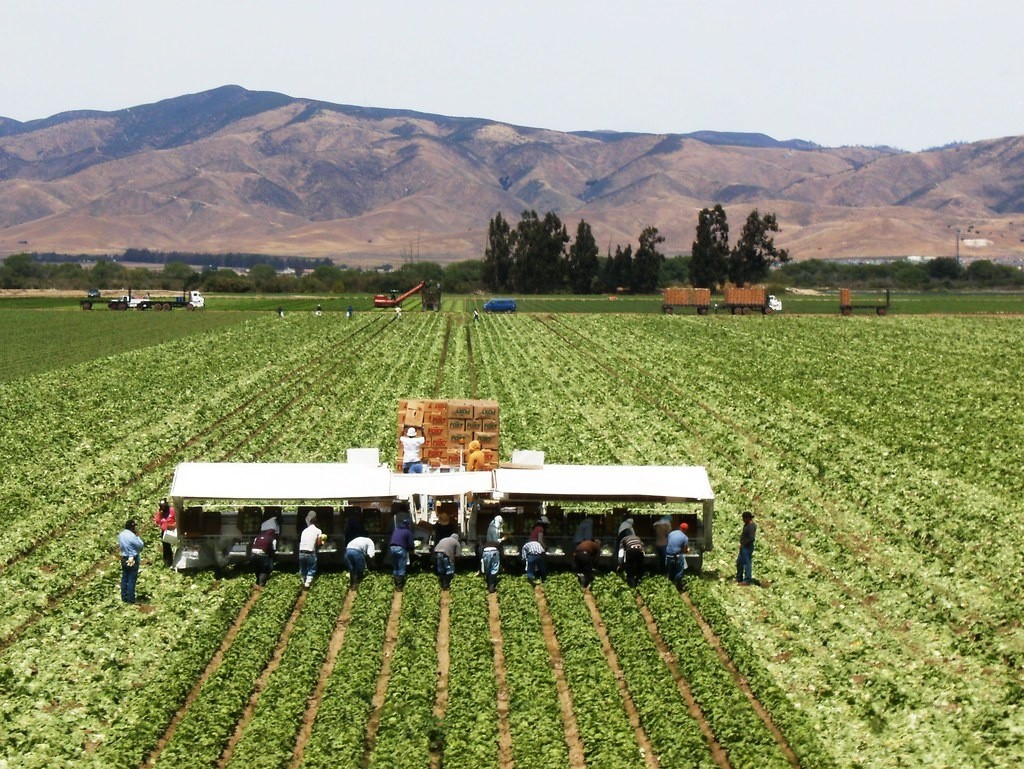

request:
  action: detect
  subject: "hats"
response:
[451,534,459,539]
[158,499,166,505]
[401,519,411,526]
[125,520,138,526]
[266,529,274,535]
[742,512,755,518]
[538,516,550,523]
[309,518,318,524]
[681,523,688,529]
[469,440,480,450]
[406,427,416,436]
[595,540,601,546]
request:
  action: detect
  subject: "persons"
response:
[466,440,486,508]
[394,306,401,317]
[389,520,414,592]
[482,515,508,594]
[472,309,479,323]
[713,304,718,315]
[298,510,322,589]
[734,512,756,586]
[278,304,282,317]
[573,518,601,588]
[616,519,644,589]
[522,516,550,588]
[317,304,322,317]
[652,515,689,591]
[400,427,425,513]
[347,305,352,317]
[155,498,176,569]
[344,537,375,588]
[251,528,277,586]
[118,520,144,604]
[432,513,461,588]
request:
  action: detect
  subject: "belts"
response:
[299,551,313,554]
[578,551,590,556]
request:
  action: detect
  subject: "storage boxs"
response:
[181,399,697,537]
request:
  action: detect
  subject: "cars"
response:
[87,288,101,298]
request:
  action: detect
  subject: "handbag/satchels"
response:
[163,529,177,544]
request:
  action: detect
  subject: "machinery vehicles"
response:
[374,280,425,308]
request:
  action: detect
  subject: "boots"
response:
[252,567,688,594]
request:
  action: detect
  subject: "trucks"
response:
[663,289,783,315]
[422,280,441,311]
[79,286,205,312]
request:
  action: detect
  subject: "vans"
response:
[483,298,516,314]
